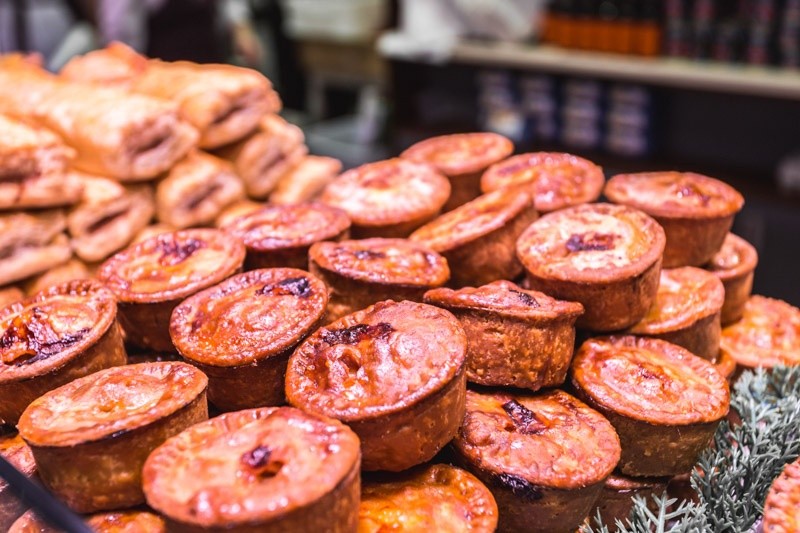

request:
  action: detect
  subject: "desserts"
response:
[0,42,800,533]
